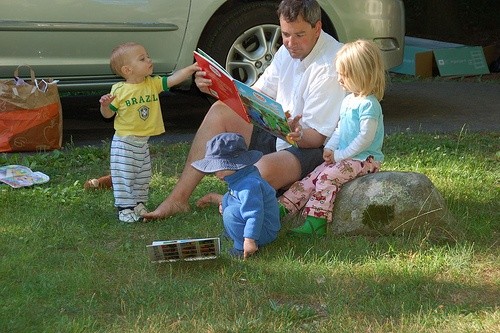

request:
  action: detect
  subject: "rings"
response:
[299,130,304,137]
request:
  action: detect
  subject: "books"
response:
[193,47,300,151]
[0,164,50,188]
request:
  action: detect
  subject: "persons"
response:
[99,40,203,223]
[189,131,281,260]
[275,38,386,237]
[141,0,352,221]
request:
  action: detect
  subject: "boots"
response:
[279,202,286,218]
[286,216,326,238]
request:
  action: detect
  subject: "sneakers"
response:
[118,203,150,222]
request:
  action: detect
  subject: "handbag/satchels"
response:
[0,64,63,153]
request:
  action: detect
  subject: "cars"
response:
[1,1,407,134]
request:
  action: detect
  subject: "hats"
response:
[191,133,263,173]
